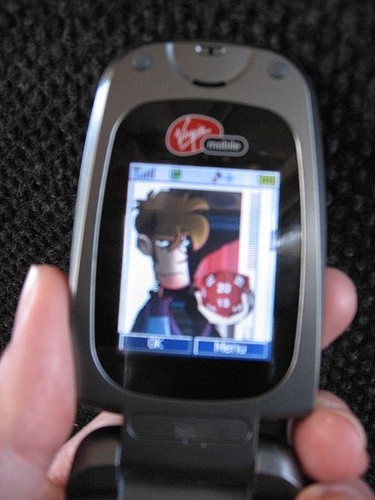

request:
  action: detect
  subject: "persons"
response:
[1,264,375,499]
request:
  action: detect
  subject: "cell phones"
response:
[63,38,328,500]
